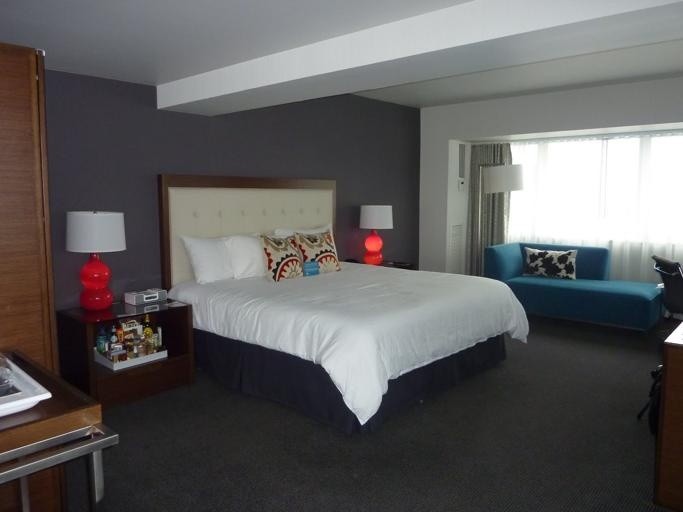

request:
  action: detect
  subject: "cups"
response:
[95,336,107,353]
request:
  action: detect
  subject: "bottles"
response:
[133,314,162,358]
[110,325,118,344]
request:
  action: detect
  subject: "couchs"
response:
[483,242,661,332]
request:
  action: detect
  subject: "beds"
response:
[160,172,509,436]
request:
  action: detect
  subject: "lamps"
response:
[359,205,393,265]
[65,210,127,311]
[477,163,523,272]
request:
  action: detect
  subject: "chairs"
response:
[650,255,683,314]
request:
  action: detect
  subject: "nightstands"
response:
[57,298,194,411]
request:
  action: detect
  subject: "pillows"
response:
[523,247,578,279]
[182,224,341,284]
